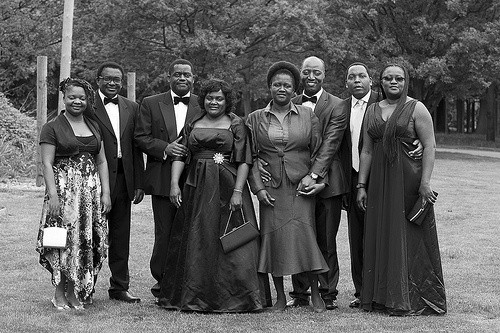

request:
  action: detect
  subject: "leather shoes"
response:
[109,289,140,303]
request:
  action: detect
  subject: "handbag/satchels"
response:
[220,207,259,254]
[43,216,67,248]
[407,191,438,226]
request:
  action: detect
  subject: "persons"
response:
[159,80,263,313]
[35,77,112,310]
[255,56,349,310]
[245,60,329,314]
[86,63,145,304]
[356,64,448,315]
[341,61,383,298]
[134,59,205,302]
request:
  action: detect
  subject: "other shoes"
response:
[321,296,337,310]
[286,298,309,307]
[349,298,361,307]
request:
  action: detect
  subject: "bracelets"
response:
[234,189,242,193]
[356,183,366,188]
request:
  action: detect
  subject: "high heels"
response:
[51,298,70,311]
[272,301,286,313]
[66,295,84,311]
[312,294,325,313]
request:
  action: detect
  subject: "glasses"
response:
[99,77,123,84]
[381,76,405,82]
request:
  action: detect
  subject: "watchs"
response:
[308,172,319,179]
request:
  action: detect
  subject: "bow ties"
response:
[104,97,118,106]
[173,97,190,105]
[302,95,318,104]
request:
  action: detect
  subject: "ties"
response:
[352,100,364,173]
